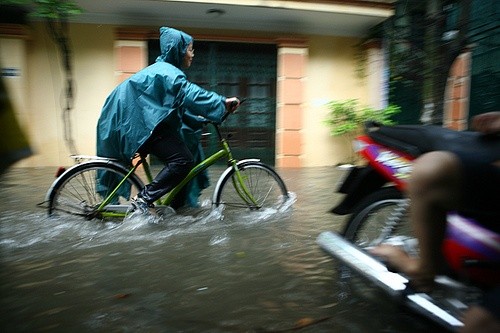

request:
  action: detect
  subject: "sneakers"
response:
[131,194,163,218]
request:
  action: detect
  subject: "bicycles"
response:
[34,96,297,231]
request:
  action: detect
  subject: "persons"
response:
[456,267,500,332]
[94,26,241,233]
[374,112,500,287]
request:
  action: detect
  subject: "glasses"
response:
[190,49,194,54]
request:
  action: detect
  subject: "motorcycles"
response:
[321,120,500,333]
